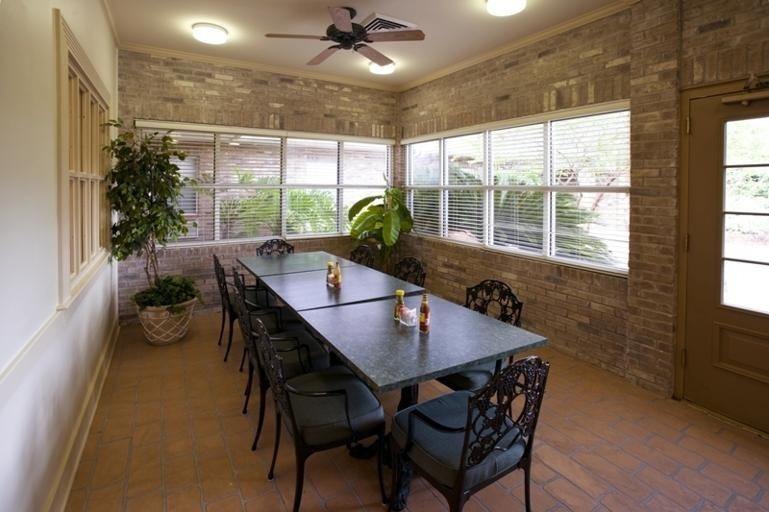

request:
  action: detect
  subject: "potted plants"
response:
[99,117,205,346]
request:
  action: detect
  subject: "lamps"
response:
[192,22,228,44]
[486,0,526,17]
[369,61,396,75]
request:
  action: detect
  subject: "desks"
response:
[235,250,550,414]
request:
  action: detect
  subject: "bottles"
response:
[334,262,342,288]
[393,289,405,320]
[418,294,430,334]
[326,262,335,287]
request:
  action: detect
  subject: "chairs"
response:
[432,278,524,420]
[349,244,375,270]
[389,355,550,510]
[254,316,390,511]
[255,239,295,256]
[390,255,427,288]
[214,254,332,452]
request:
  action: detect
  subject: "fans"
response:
[265,6,425,67]
